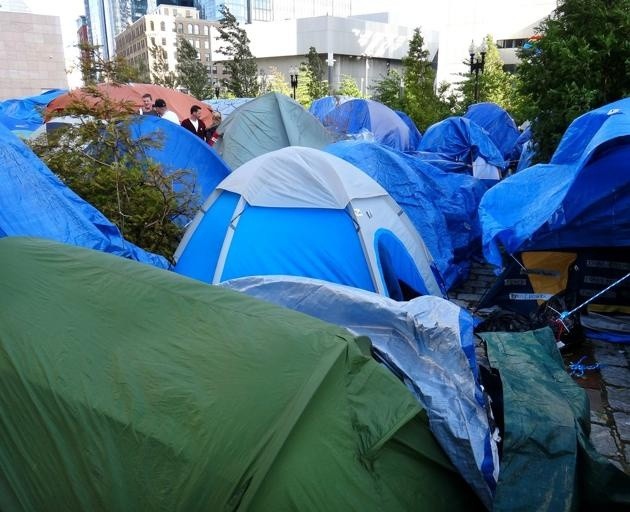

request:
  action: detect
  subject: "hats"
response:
[152,99,167,108]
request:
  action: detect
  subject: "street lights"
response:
[214,80,221,100]
[290,63,298,100]
[470,37,488,103]
